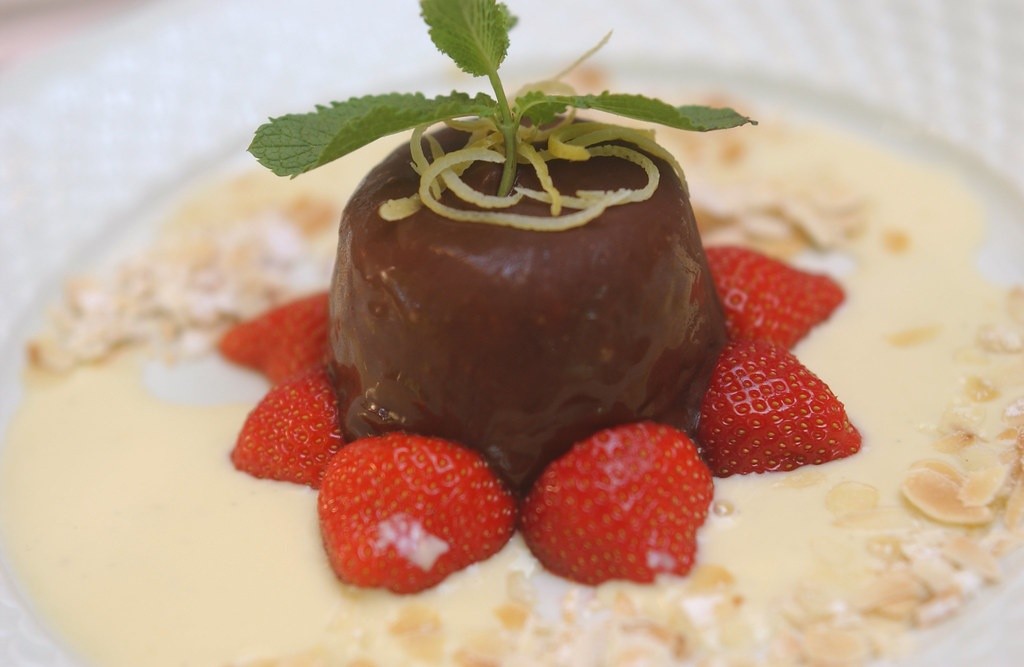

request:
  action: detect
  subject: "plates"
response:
[0,0,1024,667]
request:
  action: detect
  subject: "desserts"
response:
[11,69,1024,667]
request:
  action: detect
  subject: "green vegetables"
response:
[249,0,758,200]
